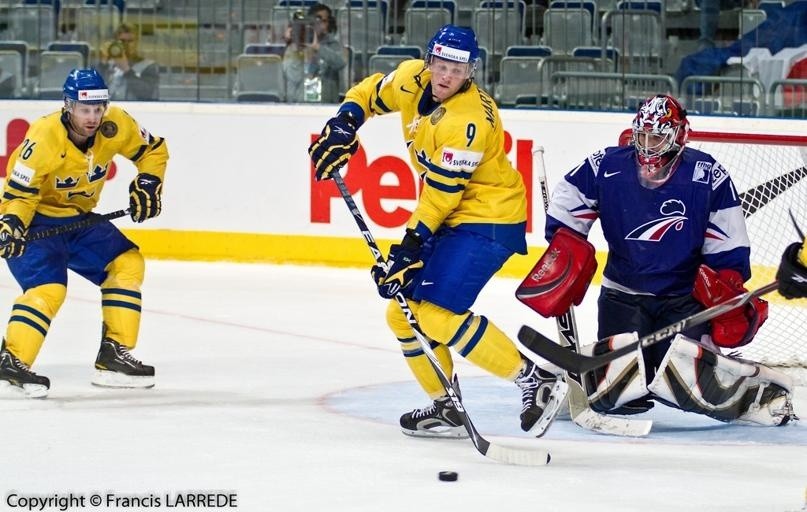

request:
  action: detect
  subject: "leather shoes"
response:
[368,244,427,298]
[128,172,164,223]
[0,214,30,260]
[307,110,362,185]
[776,239,807,302]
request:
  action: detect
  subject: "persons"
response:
[282,3,348,104]
[0,66,169,390]
[515,95,794,428]
[97,25,160,102]
[309,23,556,432]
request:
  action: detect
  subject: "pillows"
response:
[421,21,481,82]
[627,93,692,192]
[62,67,111,110]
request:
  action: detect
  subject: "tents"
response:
[325,5,337,33]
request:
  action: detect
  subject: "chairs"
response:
[510,349,559,433]
[94,320,155,377]
[396,372,467,431]
[0,0,807,119]
[0,333,51,391]
